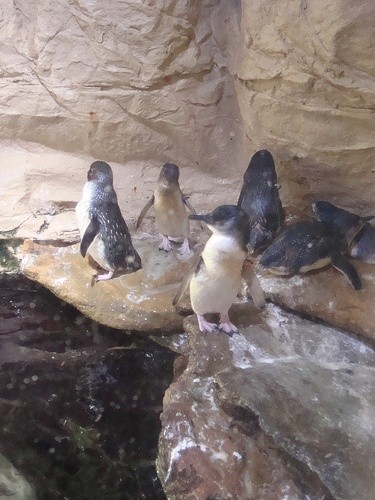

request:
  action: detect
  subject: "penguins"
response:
[173,204,267,335]
[235,148,287,257]
[74,160,144,288]
[309,200,375,264]
[134,162,204,257]
[253,219,362,292]
